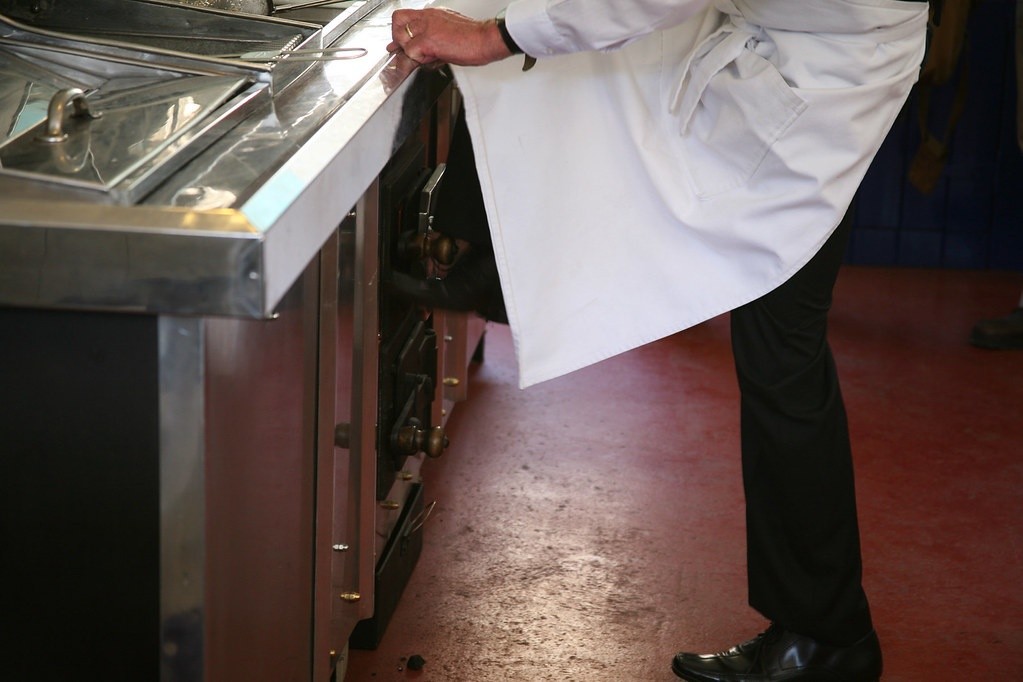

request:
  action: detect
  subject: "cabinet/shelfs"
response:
[208,78,489,682]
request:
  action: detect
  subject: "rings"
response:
[404,22,414,39]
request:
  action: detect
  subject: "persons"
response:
[969,296,1023,349]
[385,1,930,682]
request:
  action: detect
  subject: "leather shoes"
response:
[671,622,882,682]
[383,244,508,324]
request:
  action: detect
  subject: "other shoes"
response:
[969,307,1023,352]
